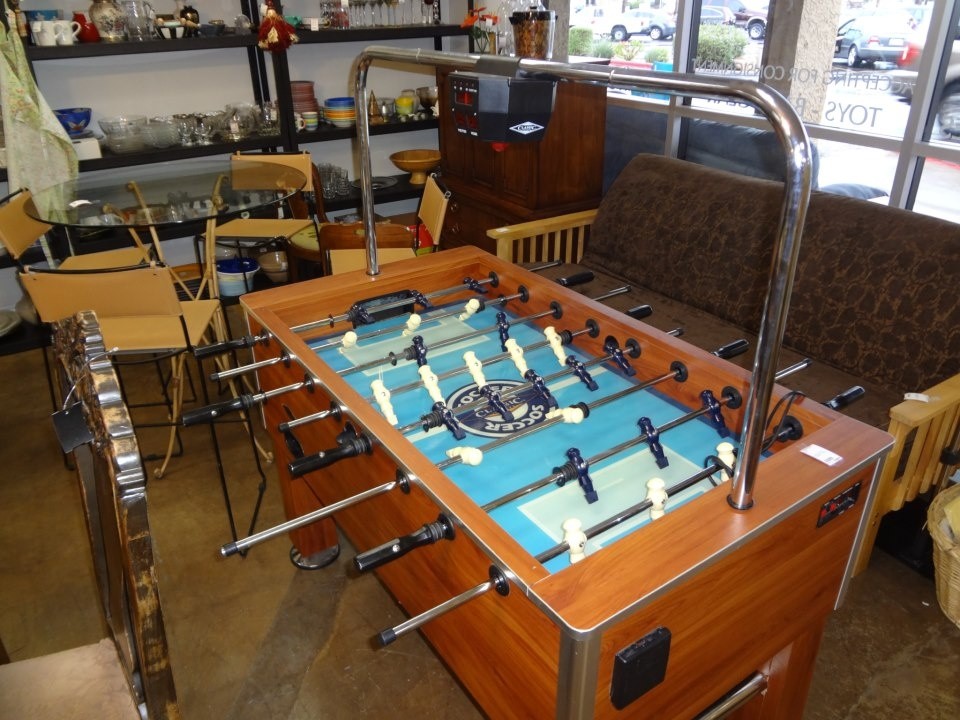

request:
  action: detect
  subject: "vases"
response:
[217,258,261,297]
[259,252,288,283]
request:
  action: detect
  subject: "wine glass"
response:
[348,0,434,28]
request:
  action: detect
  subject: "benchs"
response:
[487,152,960,577]
[602,106,888,200]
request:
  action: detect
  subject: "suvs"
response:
[701,0,768,41]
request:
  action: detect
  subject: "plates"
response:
[351,176,398,189]
[290,80,320,122]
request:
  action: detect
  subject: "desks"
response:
[24,160,307,479]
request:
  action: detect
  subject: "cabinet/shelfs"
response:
[434,66,607,264]
[22,0,474,308]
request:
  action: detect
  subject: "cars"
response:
[570,5,676,42]
[832,15,914,69]
[882,25,960,139]
[698,6,737,26]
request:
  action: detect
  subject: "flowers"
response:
[460,3,500,52]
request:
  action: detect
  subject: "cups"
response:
[318,162,350,199]
[79,214,124,230]
[293,112,305,133]
[302,112,317,130]
[121,0,150,38]
[320,0,350,30]
[54,20,80,45]
[29,21,63,46]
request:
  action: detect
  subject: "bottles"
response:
[89,0,125,39]
[496,0,546,57]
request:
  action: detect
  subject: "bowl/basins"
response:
[153,26,189,39]
[52,107,92,135]
[323,97,356,128]
[213,243,288,296]
[196,24,225,36]
[97,115,147,137]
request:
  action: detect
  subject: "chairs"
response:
[0,150,451,720]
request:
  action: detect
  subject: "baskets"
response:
[927,483,960,626]
